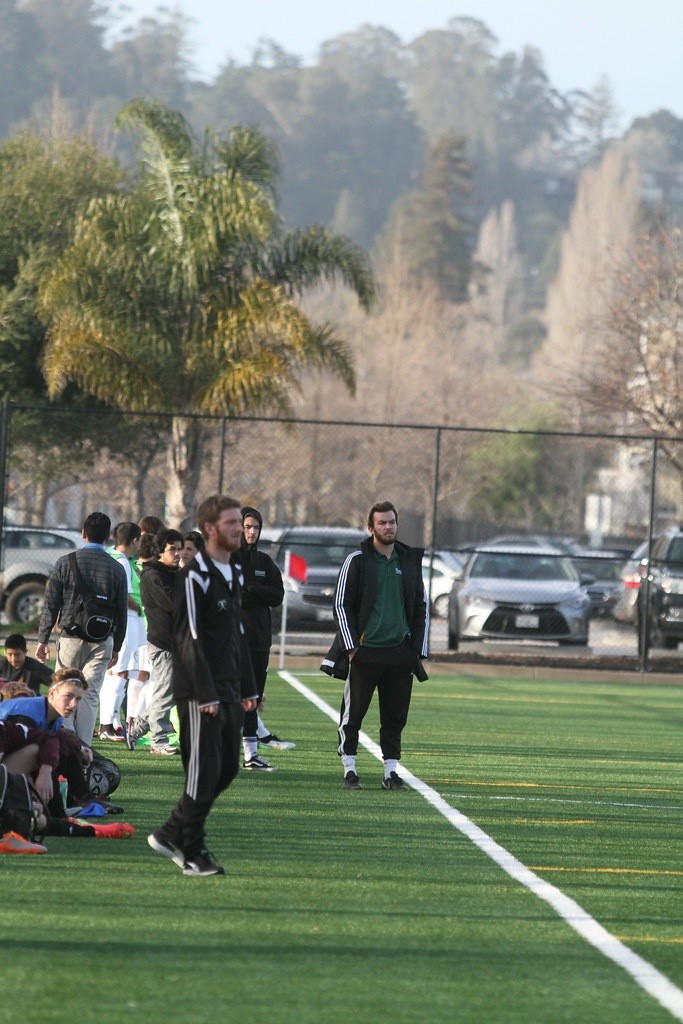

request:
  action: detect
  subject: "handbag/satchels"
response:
[70,587,117,643]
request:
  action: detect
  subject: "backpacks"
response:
[0,764,50,845]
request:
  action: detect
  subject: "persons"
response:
[256,716,297,750]
[93,521,146,742]
[36,511,127,748]
[228,506,286,771]
[146,496,259,875]
[0,634,135,854]
[320,502,429,792]
[129,516,204,755]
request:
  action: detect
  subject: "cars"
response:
[258,525,375,637]
[0,525,99,635]
[447,545,594,647]
[418,531,661,621]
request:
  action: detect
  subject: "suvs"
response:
[636,526,683,657]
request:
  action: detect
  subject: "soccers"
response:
[84,767,109,798]
[91,757,121,796]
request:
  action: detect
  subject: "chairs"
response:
[480,560,500,576]
[532,565,560,578]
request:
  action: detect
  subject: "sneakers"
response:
[242,755,277,771]
[99,729,125,742]
[262,735,296,750]
[344,770,363,789]
[149,826,184,869]
[182,848,225,875]
[85,823,135,839]
[382,771,409,790]
[0,831,49,854]
[126,716,137,750]
[105,806,124,814]
[149,745,180,755]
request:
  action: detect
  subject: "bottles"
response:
[58,775,68,809]
[30,809,38,842]
